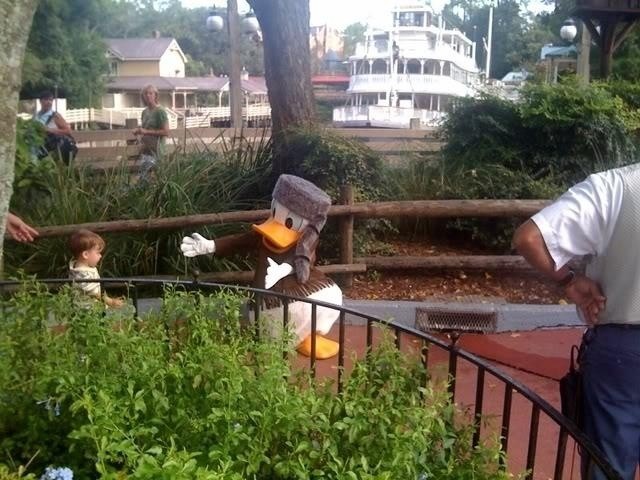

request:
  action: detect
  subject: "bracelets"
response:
[555,267,576,288]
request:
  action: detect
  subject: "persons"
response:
[6,211,39,243]
[512,160,640,479]
[30,89,71,158]
[132,83,170,189]
[65,229,125,318]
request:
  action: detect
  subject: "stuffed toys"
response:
[179,173,344,359]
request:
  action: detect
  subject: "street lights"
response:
[207,1,261,138]
[561,17,602,87]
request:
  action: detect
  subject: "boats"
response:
[332,4,523,130]
